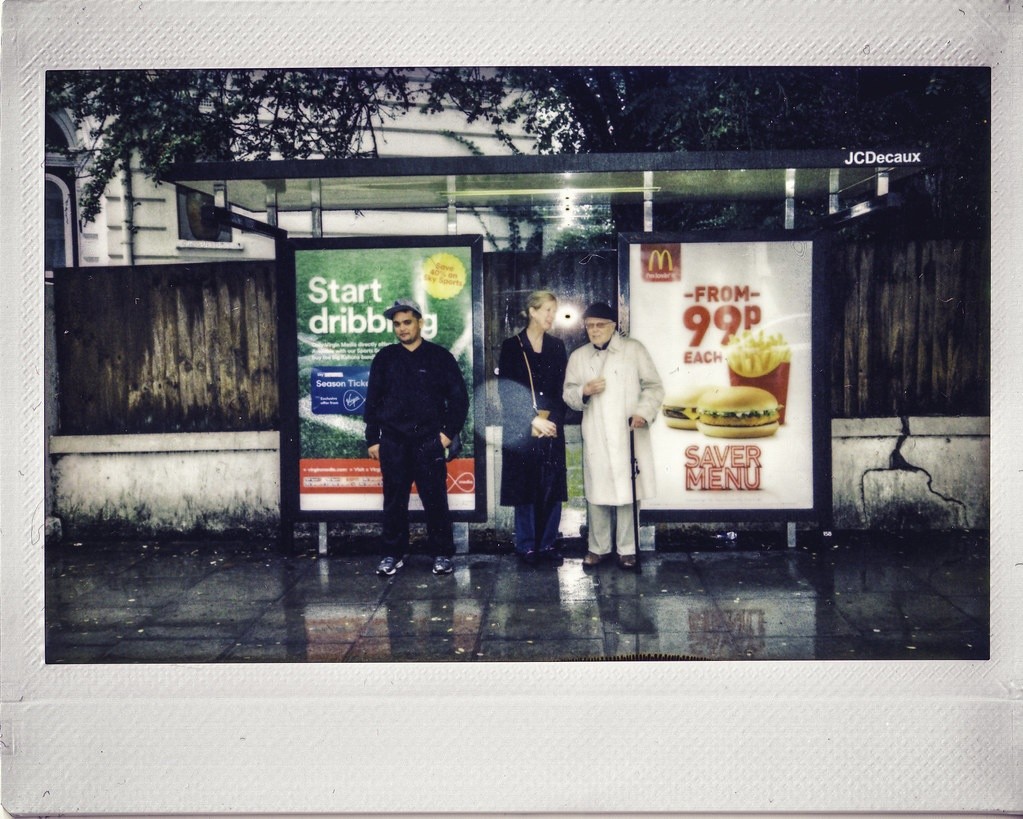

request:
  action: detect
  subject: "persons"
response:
[498,291,583,564]
[563,302,665,568]
[363,297,470,576]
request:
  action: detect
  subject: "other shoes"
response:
[523,547,563,566]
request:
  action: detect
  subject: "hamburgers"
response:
[695,385,783,438]
[661,385,716,430]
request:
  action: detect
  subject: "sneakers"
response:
[376,555,404,577]
[432,556,455,574]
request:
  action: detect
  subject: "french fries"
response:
[726,328,790,378]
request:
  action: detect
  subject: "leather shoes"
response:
[582,552,607,567]
[620,555,636,568]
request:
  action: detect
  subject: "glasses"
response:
[394,318,416,326]
[584,322,615,329]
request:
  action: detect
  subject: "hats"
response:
[383,297,423,320]
[584,302,617,321]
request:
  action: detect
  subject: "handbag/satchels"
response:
[529,408,550,437]
